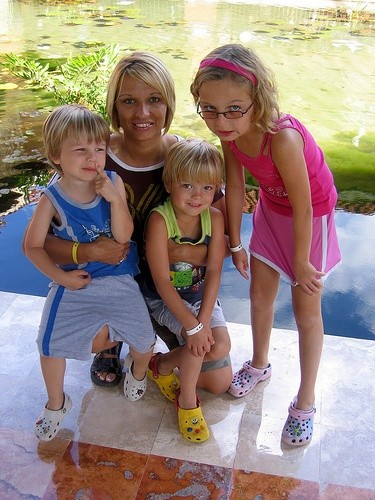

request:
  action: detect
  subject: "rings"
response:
[120,257,125,263]
[126,250,128,256]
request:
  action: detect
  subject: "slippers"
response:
[174,387,210,444]
[281,394,317,447]
[123,352,148,403]
[89,341,123,387]
[144,351,183,403]
[33,392,73,442]
[226,359,273,398]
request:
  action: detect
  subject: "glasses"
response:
[195,94,258,120]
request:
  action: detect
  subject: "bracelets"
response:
[227,241,243,253]
[185,322,204,336]
[72,242,84,265]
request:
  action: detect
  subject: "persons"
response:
[24,105,158,442]
[145,138,231,443]
[23,52,231,394]
[189,43,342,447]
